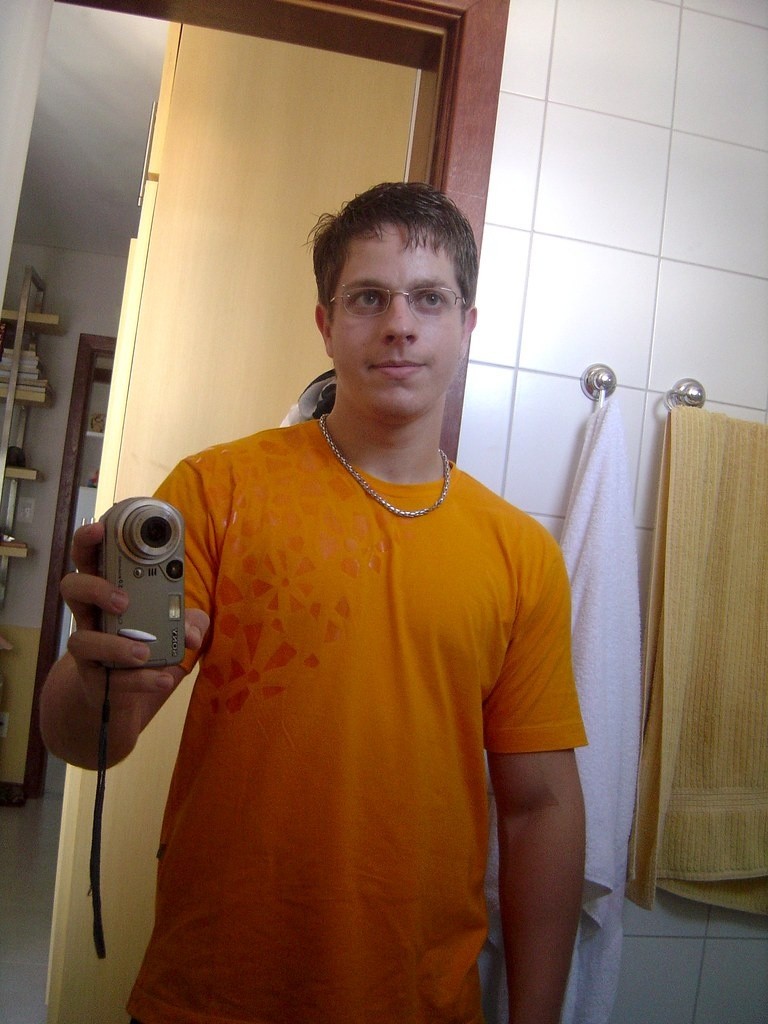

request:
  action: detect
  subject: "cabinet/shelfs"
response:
[0,263,63,609]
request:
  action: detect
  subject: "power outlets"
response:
[0,711,9,738]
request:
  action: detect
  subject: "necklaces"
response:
[320,412,451,518]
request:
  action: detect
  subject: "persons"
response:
[40,184,589,1024]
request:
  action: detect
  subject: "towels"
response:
[473,385,641,1024]
[624,399,768,928]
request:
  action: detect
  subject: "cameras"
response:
[97,495,188,668]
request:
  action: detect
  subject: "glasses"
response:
[329,286,466,319]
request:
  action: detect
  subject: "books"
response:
[0,348,54,393]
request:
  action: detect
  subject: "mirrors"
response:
[0,0,768,1024]
